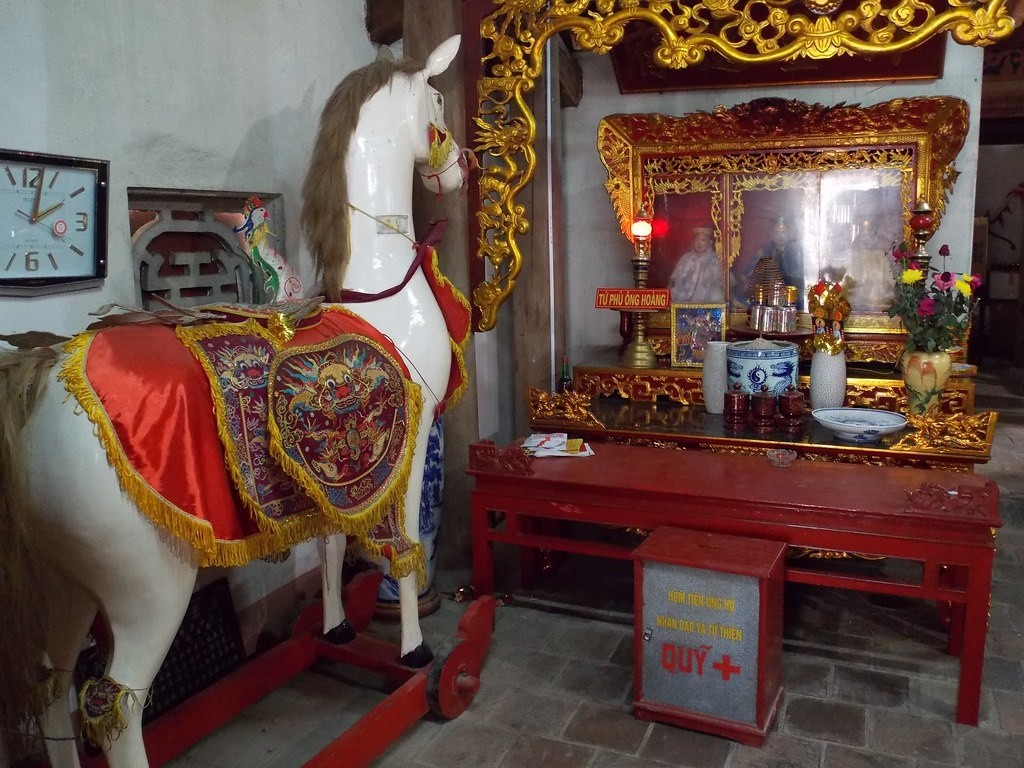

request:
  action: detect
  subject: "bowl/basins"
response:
[812,407,908,443]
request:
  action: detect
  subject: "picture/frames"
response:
[671,304,726,367]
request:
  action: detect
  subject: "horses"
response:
[0,33,469,767]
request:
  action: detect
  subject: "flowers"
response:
[884,239,981,353]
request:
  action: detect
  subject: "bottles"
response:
[558,356,573,394]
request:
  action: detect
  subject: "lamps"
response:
[621,204,657,368]
[908,194,934,293]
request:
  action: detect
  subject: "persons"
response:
[667,215,898,311]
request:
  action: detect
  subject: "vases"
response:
[810,349,845,410]
[900,349,951,415]
[701,341,730,414]
[374,416,444,621]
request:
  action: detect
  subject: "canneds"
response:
[750,282,797,333]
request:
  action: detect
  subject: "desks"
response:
[465,348,1003,726]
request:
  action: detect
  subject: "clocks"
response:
[0,149,110,297]
